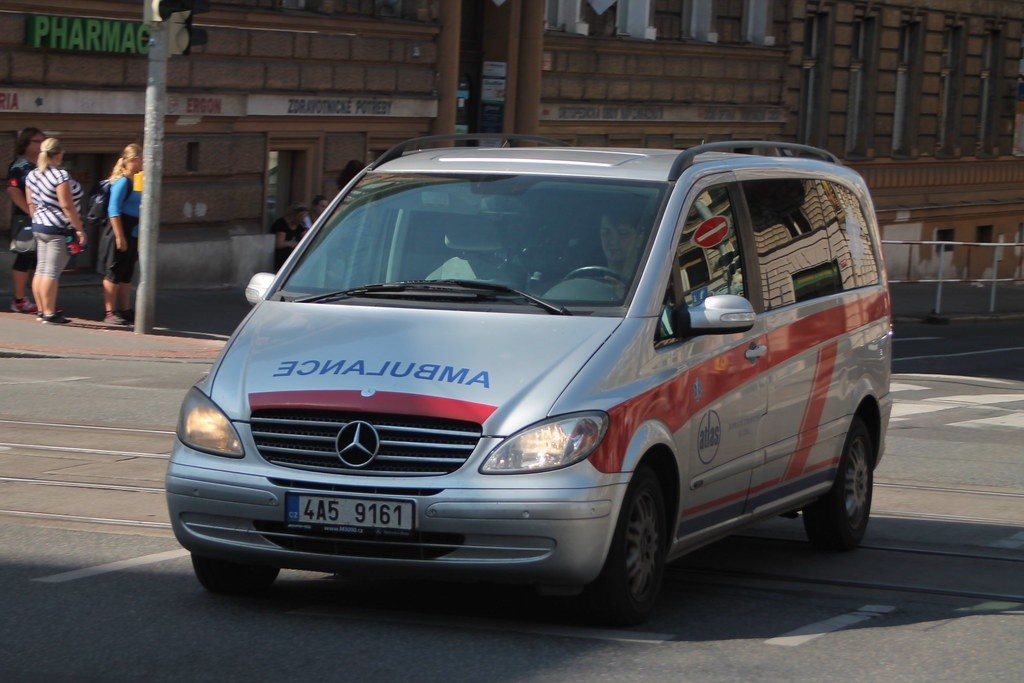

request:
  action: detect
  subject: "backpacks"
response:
[86,176,133,225]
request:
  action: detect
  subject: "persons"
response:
[6,127,143,325]
[599,199,646,298]
[269,195,329,272]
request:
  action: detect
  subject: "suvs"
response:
[163,132,893,626]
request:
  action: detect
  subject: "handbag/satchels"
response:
[9,215,36,252]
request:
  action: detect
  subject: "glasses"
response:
[30,139,43,143]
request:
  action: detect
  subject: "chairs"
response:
[423,220,526,291]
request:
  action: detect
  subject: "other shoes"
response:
[104,311,128,325]
[119,309,134,321]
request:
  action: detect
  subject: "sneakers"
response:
[11,296,37,313]
[56,306,64,312]
[43,310,71,325]
[36,313,44,321]
